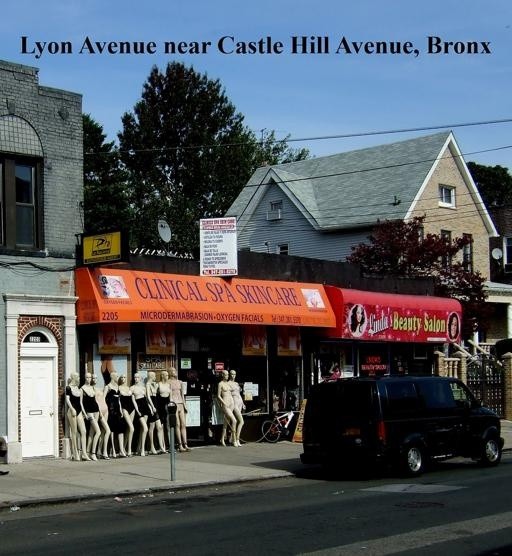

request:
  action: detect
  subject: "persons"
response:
[447,312,460,339]
[229,370,246,447]
[101,276,107,283]
[350,304,366,333]
[216,370,239,448]
[64,367,191,462]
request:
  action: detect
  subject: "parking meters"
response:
[168,401,180,481]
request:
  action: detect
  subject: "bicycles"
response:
[257,394,298,441]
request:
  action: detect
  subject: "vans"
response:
[300,371,507,478]
